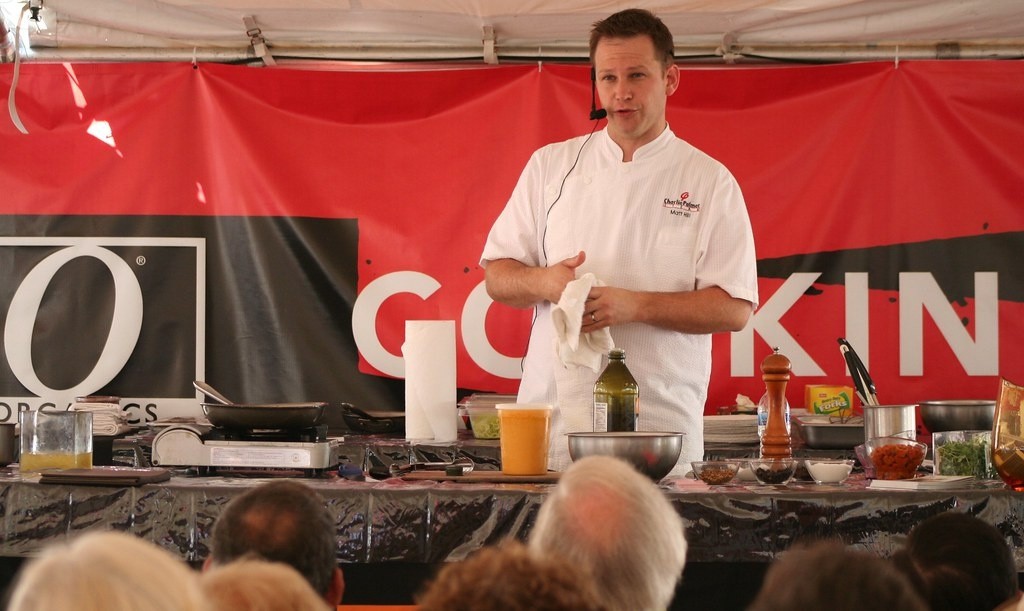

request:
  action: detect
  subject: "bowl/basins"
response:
[564,430,684,487]
[854,436,927,481]
[461,392,518,439]
[748,460,798,485]
[803,460,856,485]
[690,461,742,485]
[726,458,759,481]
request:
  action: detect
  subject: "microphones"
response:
[590,67,607,121]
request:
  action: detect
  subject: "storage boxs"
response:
[933,431,996,479]
[459,408,501,440]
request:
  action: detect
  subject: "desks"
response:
[0,467,1024,610]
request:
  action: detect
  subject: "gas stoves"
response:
[150,420,340,477]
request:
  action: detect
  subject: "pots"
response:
[918,400,996,434]
[338,401,404,435]
[199,401,330,432]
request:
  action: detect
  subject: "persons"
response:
[480,10,761,483]
[895,510,1024,611]
[424,535,614,611]
[4,525,213,611]
[200,478,345,611]
[746,537,929,611]
[527,449,688,611]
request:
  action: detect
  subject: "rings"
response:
[591,313,596,322]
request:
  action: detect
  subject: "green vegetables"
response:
[936,437,998,480]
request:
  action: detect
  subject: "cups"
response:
[19,409,93,472]
[931,430,991,476]
[991,377,1024,491]
[0,422,16,464]
[859,404,919,443]
[496,403,555,477]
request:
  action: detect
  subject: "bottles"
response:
[757,383,792,463]
[593,348,641,434]
